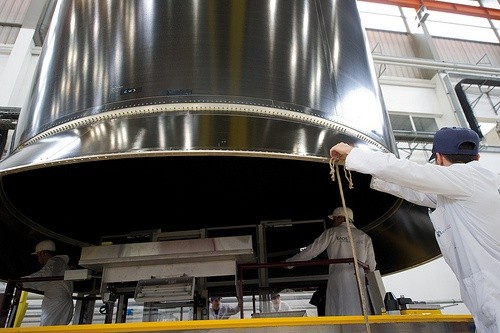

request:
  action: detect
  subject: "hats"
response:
[30,239,56,255]
[429,126,480,162]
[210,292,222,301]
[328,207,355,222]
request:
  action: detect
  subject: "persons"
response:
[282,207,376,316]
[264,293,291,313]
[21,240,73,326]
[329,127,500,333]
[209,296,240,320]
